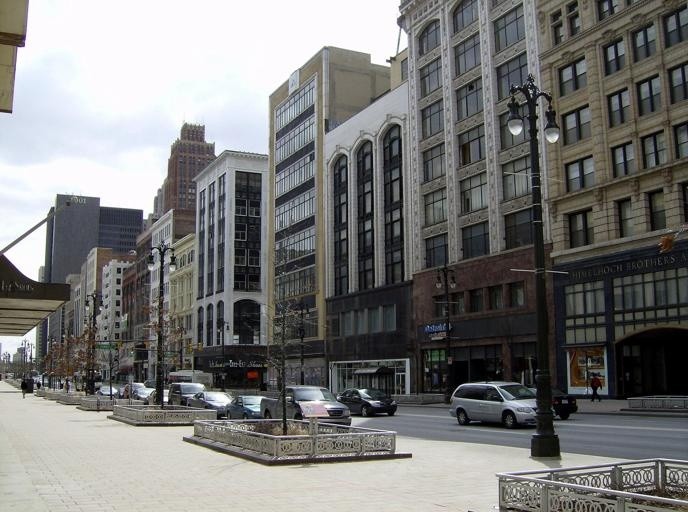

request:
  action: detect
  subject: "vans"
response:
[167,383,207,406]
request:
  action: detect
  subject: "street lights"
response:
[217,320,232,393]
[62,327,75,393]
[505,73,561,458]
[436,265,457,406]
[47,336,56,389]
[147,240,177,405]
[294,301,311,386]
[21,338,35,379]
[84,290,104,396]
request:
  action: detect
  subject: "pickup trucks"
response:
[260,383,351,434]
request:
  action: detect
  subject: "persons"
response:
[122,385,129,399]
[589,374,602,402]
[21,378,28,398]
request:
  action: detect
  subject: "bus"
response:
[75,374,102,391]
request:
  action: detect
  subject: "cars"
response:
[225,395,266,419]
[95,385,118,396]
[336,386,398,417]
[449,382,578,429]
[117,379,169,405]
[186,391,233,420]
